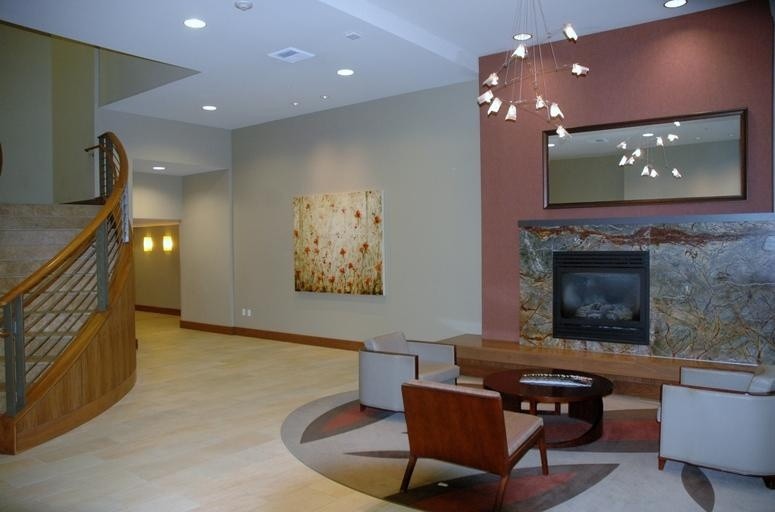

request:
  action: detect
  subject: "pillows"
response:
[747,365,775,392]
[363,331,410,354]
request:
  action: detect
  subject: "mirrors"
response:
[542,108,747,208]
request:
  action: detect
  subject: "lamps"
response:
[615,121,683,179]
[478,0,589,137]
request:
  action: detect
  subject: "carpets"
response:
[281,382,775,512]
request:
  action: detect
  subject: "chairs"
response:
[359,331,460,412]
[400,380,549,512]
[656,365,775,489]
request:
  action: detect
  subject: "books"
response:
[519,373,594,388]
